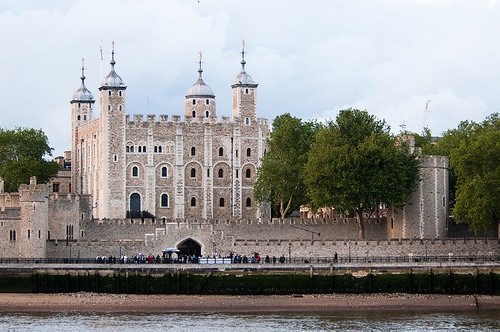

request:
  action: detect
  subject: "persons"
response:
[94,250,339,264]
